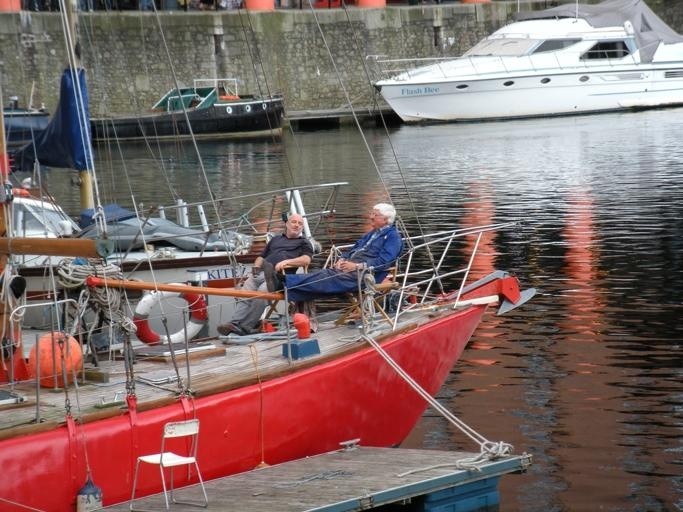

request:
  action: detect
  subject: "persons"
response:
[262,203,403,302]
[216,213,313,336]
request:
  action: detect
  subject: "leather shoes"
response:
[263,259,279,292]
[217,323,246,336]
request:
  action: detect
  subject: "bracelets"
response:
[355,263,359,270]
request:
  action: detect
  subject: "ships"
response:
[1,181,348,331]
[1,264,534,511]
[372,0,682,122]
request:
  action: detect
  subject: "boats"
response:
[77,76,283,143]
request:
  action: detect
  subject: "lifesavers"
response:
[13,188,30,197]
[134,283,207,344]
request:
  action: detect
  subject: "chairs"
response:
[251,259,308,320]
[332,258,400,327]
[128,420,210,512]
